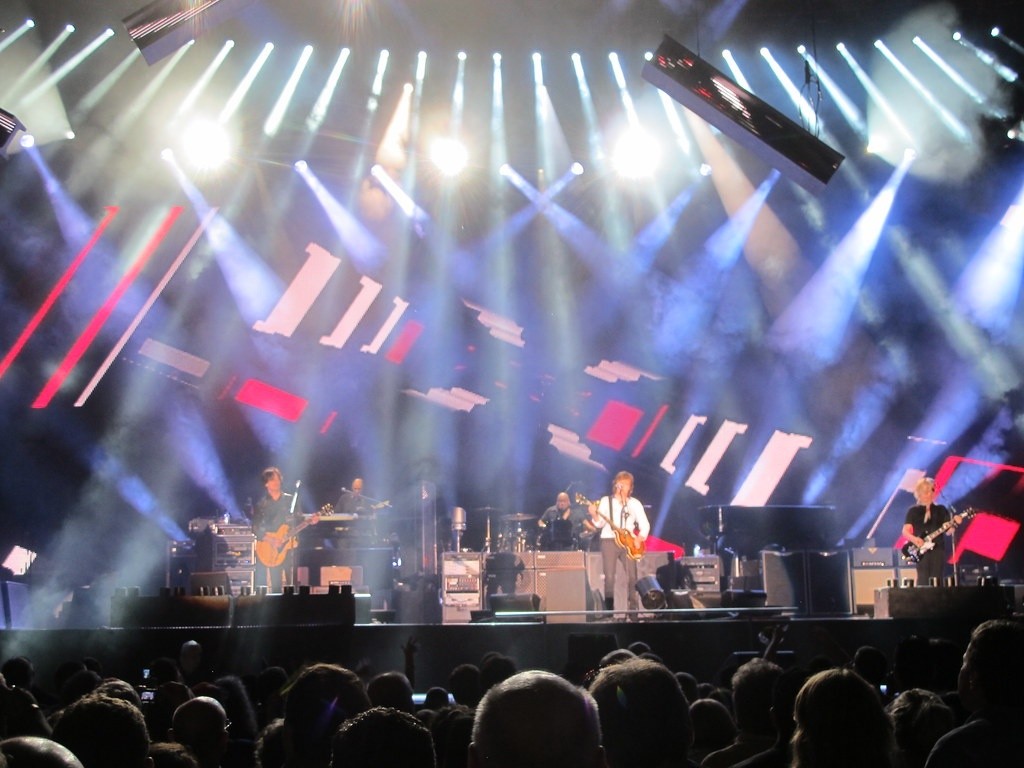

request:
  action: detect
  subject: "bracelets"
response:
[543,525,546,527]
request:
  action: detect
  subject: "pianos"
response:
[698,503,840,617]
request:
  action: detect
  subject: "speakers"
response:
[190,572,232,595]
[481,551,675,624]
[758,549,1015,623]
[319,566,364,588]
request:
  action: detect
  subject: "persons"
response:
[539,493,596,551]
[902,478,963,585]
[0,615,1024,768]
[336,478,368,513]
[589,471,651,621]
[253,466,317,594]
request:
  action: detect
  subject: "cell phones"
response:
[144,668,150,679]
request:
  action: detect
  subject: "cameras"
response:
[136,689,158,705]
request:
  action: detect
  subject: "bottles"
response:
[224,510,230,524]
[694,545,700,557]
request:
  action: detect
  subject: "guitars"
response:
[254,501,335,568]
[901,505,977,567]
[572,489,646,560]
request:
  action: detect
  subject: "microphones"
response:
[616,483,623,490]
[341,487,345,491]
[296,480,302,489]
[932,484,936,491]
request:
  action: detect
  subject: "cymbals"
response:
[474,507,502,512]
[505,514,537,522]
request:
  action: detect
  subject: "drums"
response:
[548,519,573,543]
